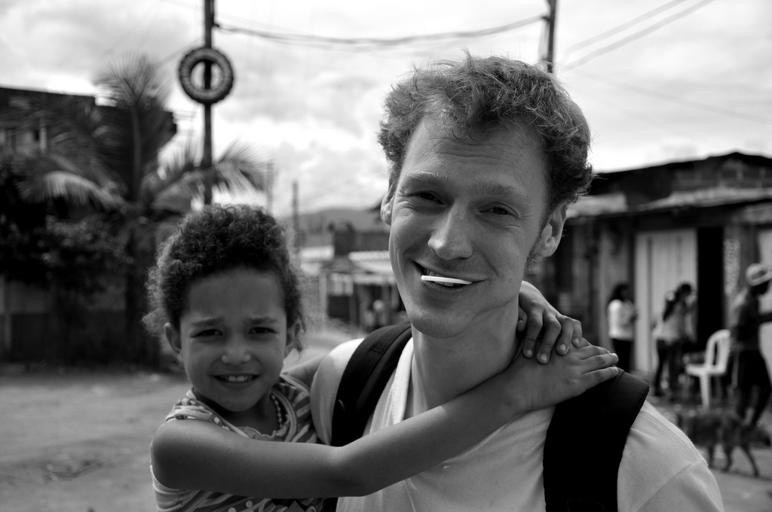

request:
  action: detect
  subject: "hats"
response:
[747,263,771,287]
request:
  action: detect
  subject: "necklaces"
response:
[271,394,283,426]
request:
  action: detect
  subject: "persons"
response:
[309,55,722,510]
[727,262,772,428]
[141,202,624,512]
[606,283,636,374]
[653,283,698,397]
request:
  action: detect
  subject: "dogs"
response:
[672,406,761,477]
